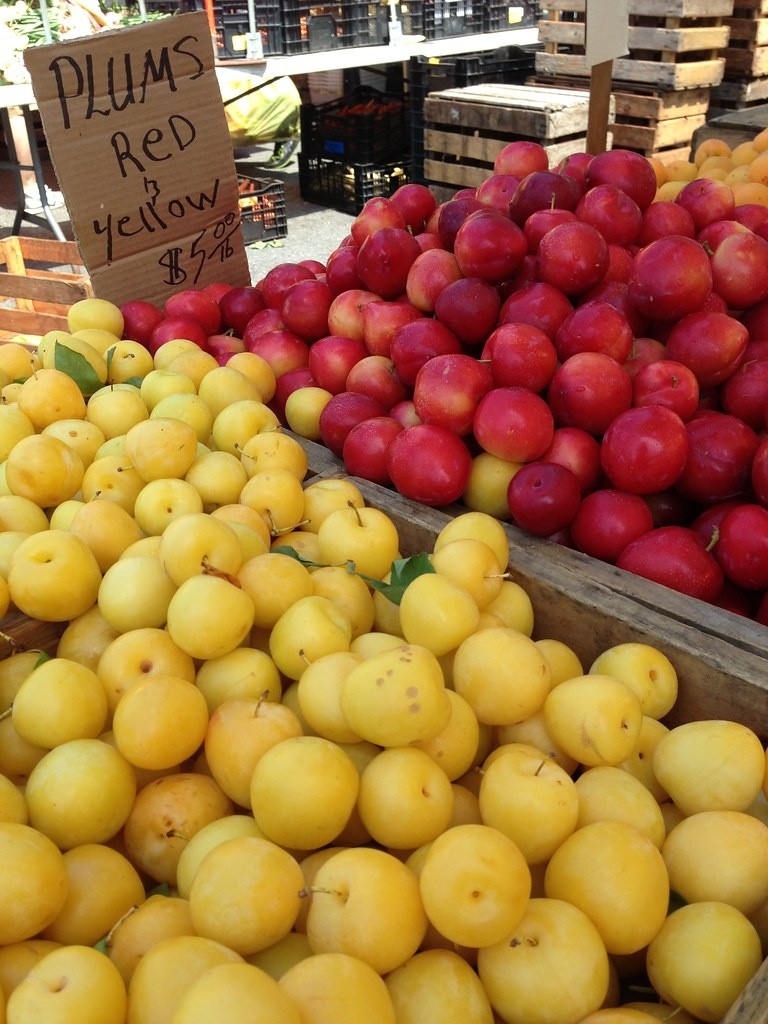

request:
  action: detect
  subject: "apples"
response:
[0,296,768,1024]
[112,126,768,621]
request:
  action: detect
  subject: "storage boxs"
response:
[423,81,615,207]
[533,0,734,93]
[236,173,288,246]
[0,237,94,353]
[395,0,485,43]
[405,45,537,131]
[409,127,504,189]
[524,73,710,166]
[484,0,540,34]
[297,152,416,217]
[280,0,391,56]
[681,0,768,109]
[299,85,410,164]
[124,0,284,60]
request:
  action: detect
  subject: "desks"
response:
[0,84,81,274]
